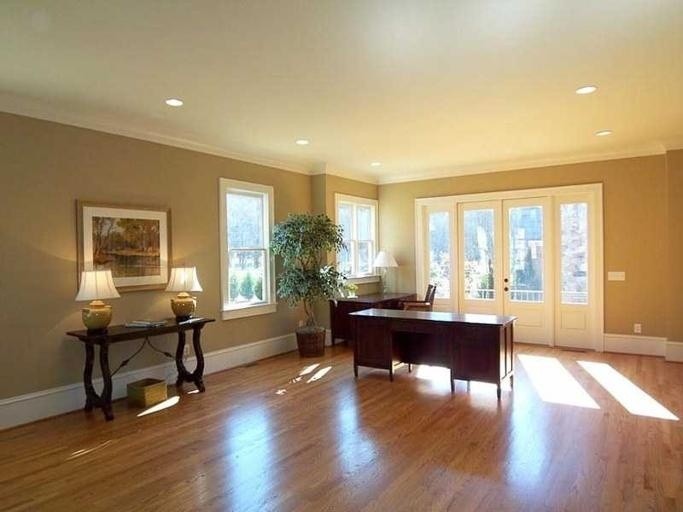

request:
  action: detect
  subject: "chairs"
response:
[398,284,436,311]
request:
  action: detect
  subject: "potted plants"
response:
[268,212,349,357]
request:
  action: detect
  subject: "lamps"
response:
[165,265,203,317]
[75,268,121,330]
[374,249,398,293]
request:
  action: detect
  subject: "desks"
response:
[349,307,518,400]
[328,291,417,346]
[65,315,216,421]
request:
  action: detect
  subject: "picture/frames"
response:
[74,198,173,294]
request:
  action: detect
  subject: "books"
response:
[125,320,168,327]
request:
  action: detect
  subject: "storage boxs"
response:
[126,377,168,408]
